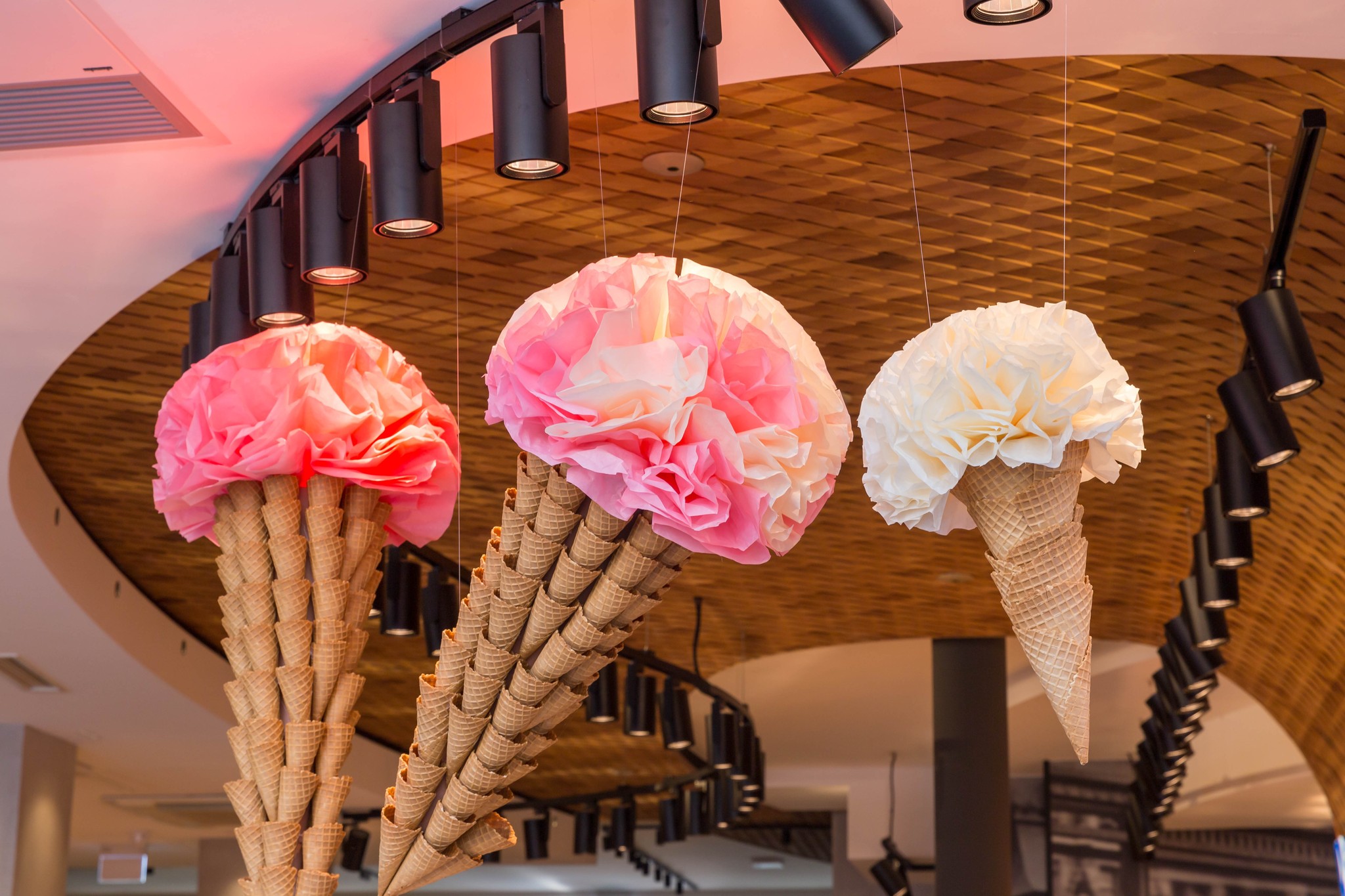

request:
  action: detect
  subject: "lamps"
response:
[631,1,724,124]
[963,0,1053,27]
[1126,272,1325,858]
[367,73,444,240]
[490,2,570,178]
[340,549,765,894]
[870,851,913,896]
[181,179,314,378]
[778,1,904,80]
[300,128,367,287]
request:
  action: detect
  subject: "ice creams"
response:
[151,320,459,896]
[856,301,1144,765]
[376,252,853,896]
